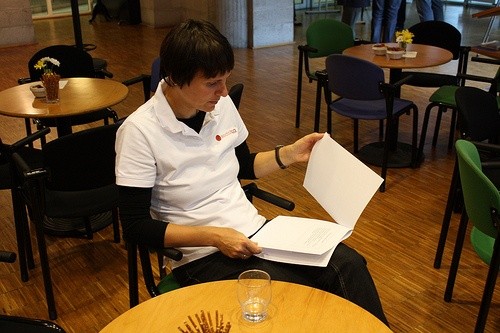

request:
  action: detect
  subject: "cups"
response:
[44,84,59,103]
[237,270,272,323]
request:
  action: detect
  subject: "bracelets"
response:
[275,145,286,169]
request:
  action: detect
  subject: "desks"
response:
[0,77,129,237]
[343,43,453,169]
[99,279,394,333]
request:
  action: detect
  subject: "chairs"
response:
[295,19,470,193]
[433,86,500,333]
[0,44,296,333]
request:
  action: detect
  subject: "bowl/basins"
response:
[372,46,386,55]
[29,85,46,97]
[386,50,404,59]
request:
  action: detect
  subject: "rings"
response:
[242,255,246,259]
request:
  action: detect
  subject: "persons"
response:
[114,18,389,327]
[337,0,370,41]
[371,0,401,43]
[416,0,444,23]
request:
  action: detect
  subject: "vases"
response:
[44,82,60,103]
[398,42,407,52]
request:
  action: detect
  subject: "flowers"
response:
[395,29,413,44]
[33,56,61,85]
